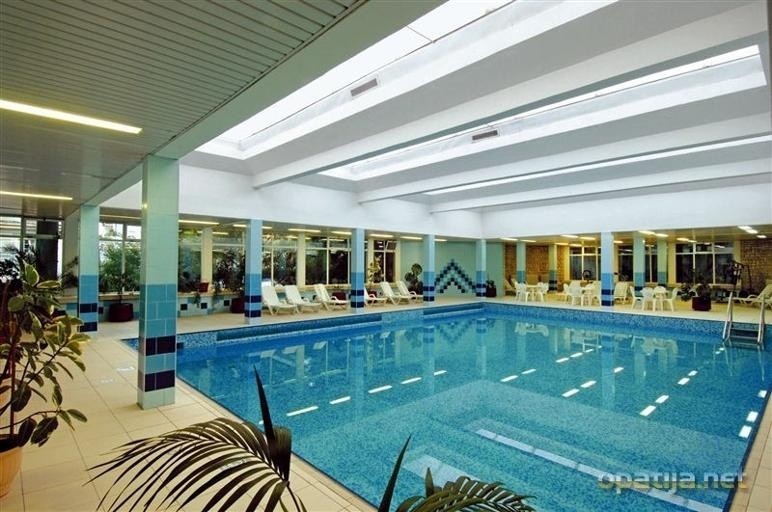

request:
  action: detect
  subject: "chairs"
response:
[314,283,351,312]
[396,281,423,305]
[726,282,772,310]
[262,285,298,316]
[380,281,412,305]
[364,286,387,307]
[555,280,681,312]
[285,285,322,314]
[504,277,549,303]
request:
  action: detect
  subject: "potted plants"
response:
[485,279,496,297]
[365,256,382,303]
[689,268,712,312]
[332,283,346,305]
[0,254,91,498]
